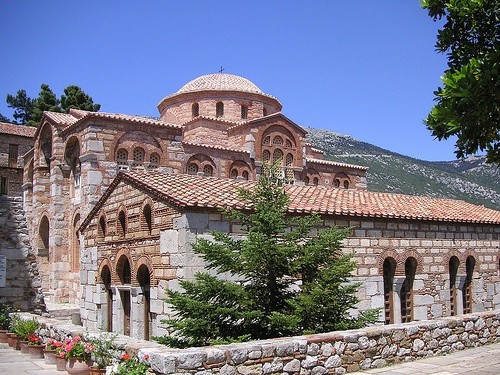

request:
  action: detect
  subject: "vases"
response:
[28,344,94,375]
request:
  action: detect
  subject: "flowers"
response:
[109,353,151,375]
[25,323,96,360]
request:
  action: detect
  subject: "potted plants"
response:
[90,327,127,375]
[0,301,40,354]
